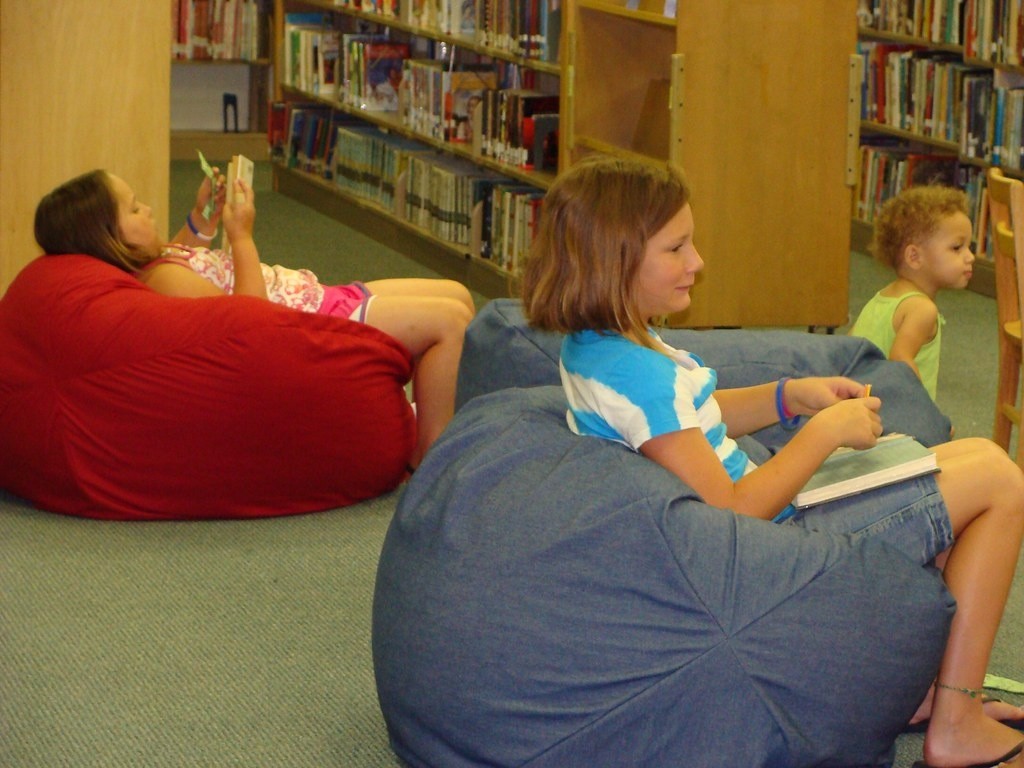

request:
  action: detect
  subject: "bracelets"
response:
[776,377,801,430]
[187,216,218,241]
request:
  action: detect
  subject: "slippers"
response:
[912,740,1024,768]
[901,686,1024,733]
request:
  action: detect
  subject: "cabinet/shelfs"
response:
[1,0,1024,333]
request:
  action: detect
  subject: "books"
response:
[223,153,254,255]
[855,0,1024,259]
[791,432,940,509]
[173,0,561,275]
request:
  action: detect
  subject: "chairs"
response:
[986,167,1024,470]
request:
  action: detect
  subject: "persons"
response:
[34,167,476,483]
[523,153,1024,768]
[846,184,975,437]
